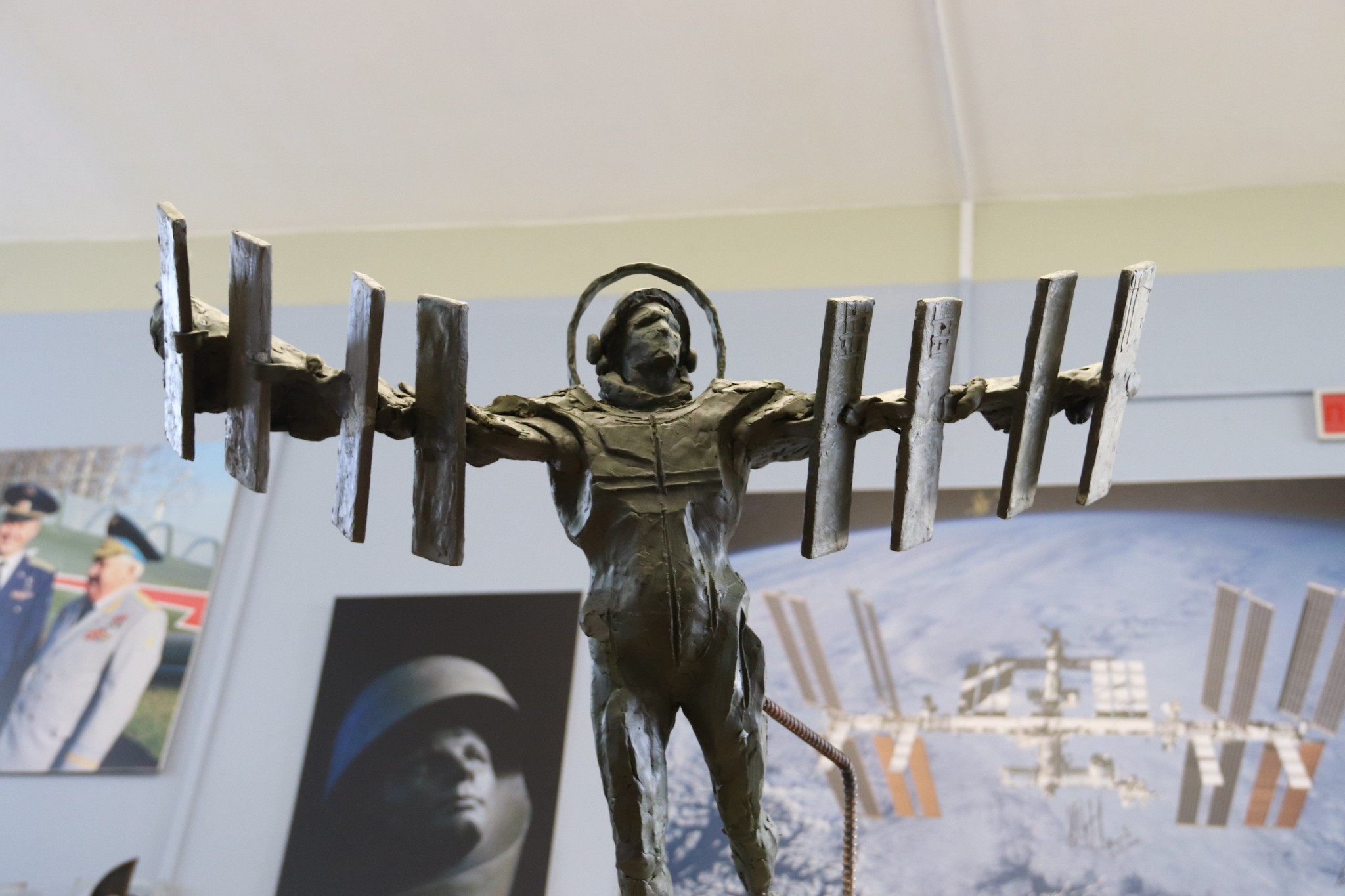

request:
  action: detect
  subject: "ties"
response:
[77,601,94,623]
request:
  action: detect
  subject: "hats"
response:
[5,483,58,525]
[94,510,164,566]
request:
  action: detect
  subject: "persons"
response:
[0,484,168,774]
[317,657,534,896]
[146,262,1140,896]
